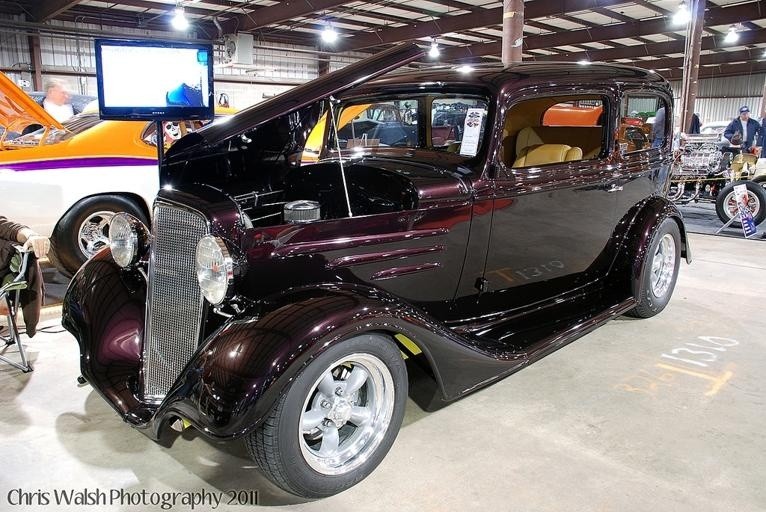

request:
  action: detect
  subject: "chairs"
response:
[0,242,34,375]
[510,141,584,173]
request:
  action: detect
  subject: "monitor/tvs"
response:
[94,38,213,120]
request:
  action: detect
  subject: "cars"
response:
[0,86,98,142]
[0,69,414,275]
[696,119,733,139]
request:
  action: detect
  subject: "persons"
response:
[163,121,187,154]
[0,215,51,259]
[43,77,75,125]
[651,107,666,147]
[723,106,762,154]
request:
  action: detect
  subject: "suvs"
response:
[60,39,695,504]
[668,132,765,229]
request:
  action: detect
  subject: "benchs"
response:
[514,125,602,160]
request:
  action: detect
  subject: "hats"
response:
[740,106,749,112]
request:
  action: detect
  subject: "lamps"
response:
[428,38,440,58]
[725,25,739,43]
[169,0,188,31]
[674,0,691,25]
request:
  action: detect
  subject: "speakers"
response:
[224,34,254,65]
